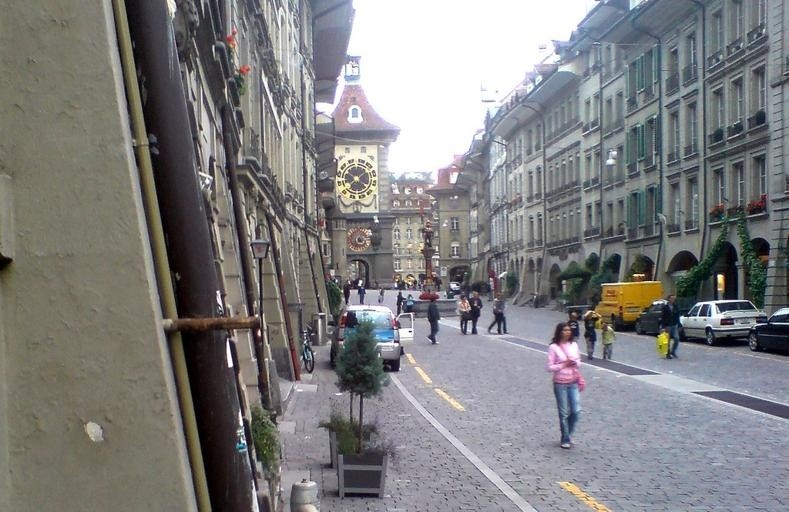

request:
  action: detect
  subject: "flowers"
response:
[227,23,250,96]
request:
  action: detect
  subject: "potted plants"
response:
[316,319,400,498]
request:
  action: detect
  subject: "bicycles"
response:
[300,325,316,374]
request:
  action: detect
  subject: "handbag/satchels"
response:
[463,311,474,320]
[577,374,585,391]
[657,332,669,355]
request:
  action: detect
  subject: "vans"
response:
[591,281,664,331]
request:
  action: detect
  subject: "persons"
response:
[343,280,351,305]
[397,292,406,315]
[601,322,616,360]
[394,274,442,294]
[581,307,601,361]
[662,295,680,359]
[446,284,509,335]
[426,299,440,344]
[358,284,366,304]
[377,285,385,304]
[422,221,433,247]
[568,312,580,337]
[546,323,582,449]
[407,294,414,313]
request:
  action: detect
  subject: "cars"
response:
[448,282,460,290]
[634,298,788,356]
[326,305,414,372]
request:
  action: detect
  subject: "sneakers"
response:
[561,436,577,449]
[666,352,677,359]
